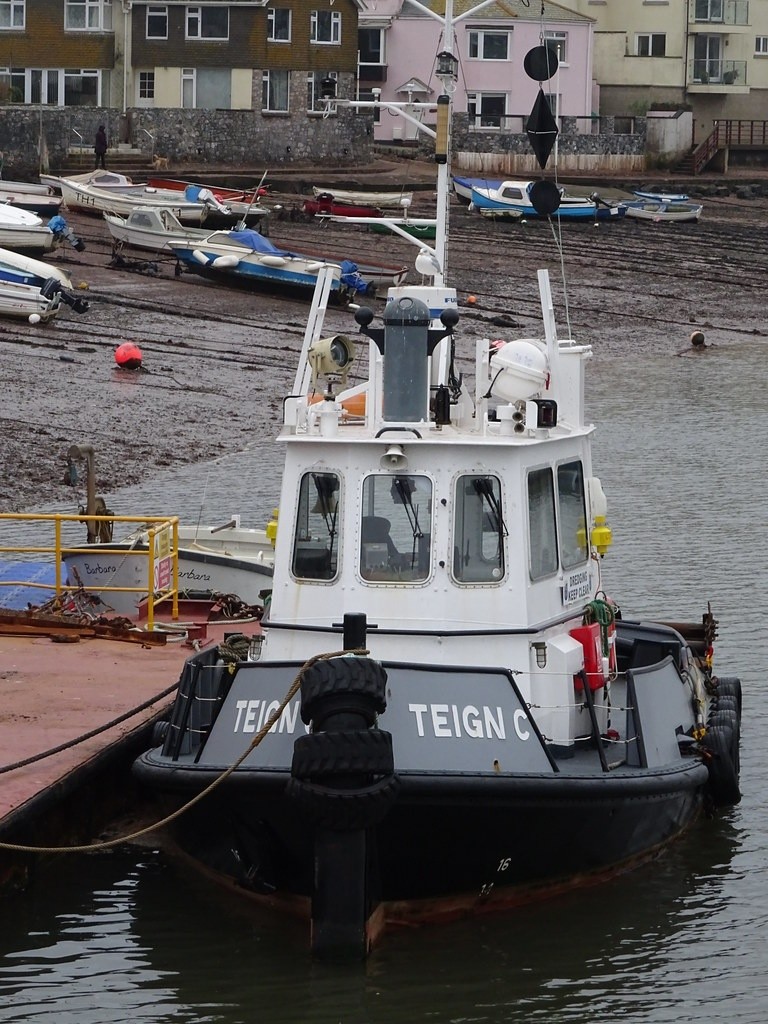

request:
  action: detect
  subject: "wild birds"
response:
[413,246,446,287]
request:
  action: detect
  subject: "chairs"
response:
[362,516,410,575]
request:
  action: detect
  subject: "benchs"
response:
[656,205,666,213]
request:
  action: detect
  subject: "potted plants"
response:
[701,71,711,85]
[724,68,738,84]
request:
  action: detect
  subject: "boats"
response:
[219,200,270,231]
[39,170,148,195]
[166,170,410,299]
[631,191,691,204]
[148,175,273,203]
[103,204,217,255]
[0,202,44,229]
[0,190,64,218]
[0,247,90,326]
[0,214,87,257]
[609,202,703,223]
[59,176,232,230]
[369,220,437,238]
[129,0,745,931]
[452,174,501,206]
[301,193,387,217]
[0,180,56,199]
[469,180,630,221]
[313,186,415,210]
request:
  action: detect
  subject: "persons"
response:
[94,125,108,170]
